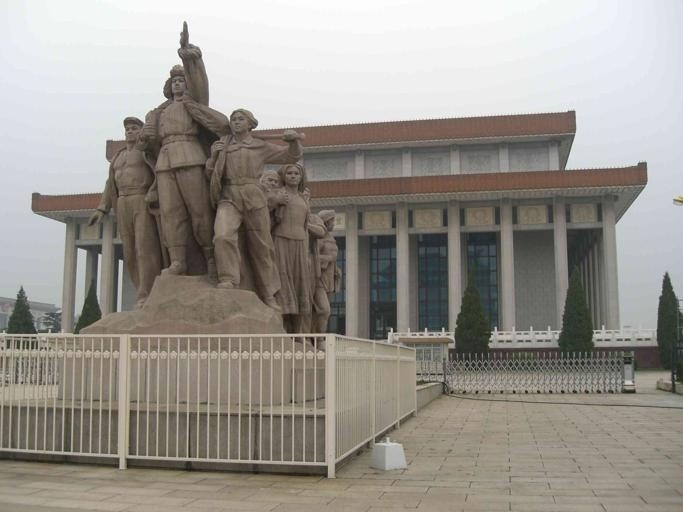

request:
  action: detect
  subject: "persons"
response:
[90,116,164,309]
[311,209,338,348]
[263,163,326,348]
[138,20,218,279]
[205,109,304,311]
